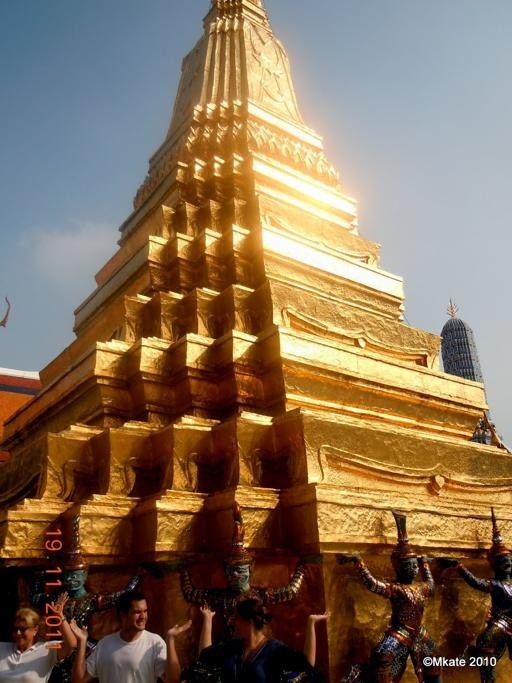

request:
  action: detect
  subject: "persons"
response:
[439,507,512,683]
[0,591,77,683]
[70,590,193,683]
[339,511,441,682]
[198,598,324,682]
[471,417,492,445]
[175,502,319,681]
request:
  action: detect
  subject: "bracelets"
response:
[58,618,66,622]
